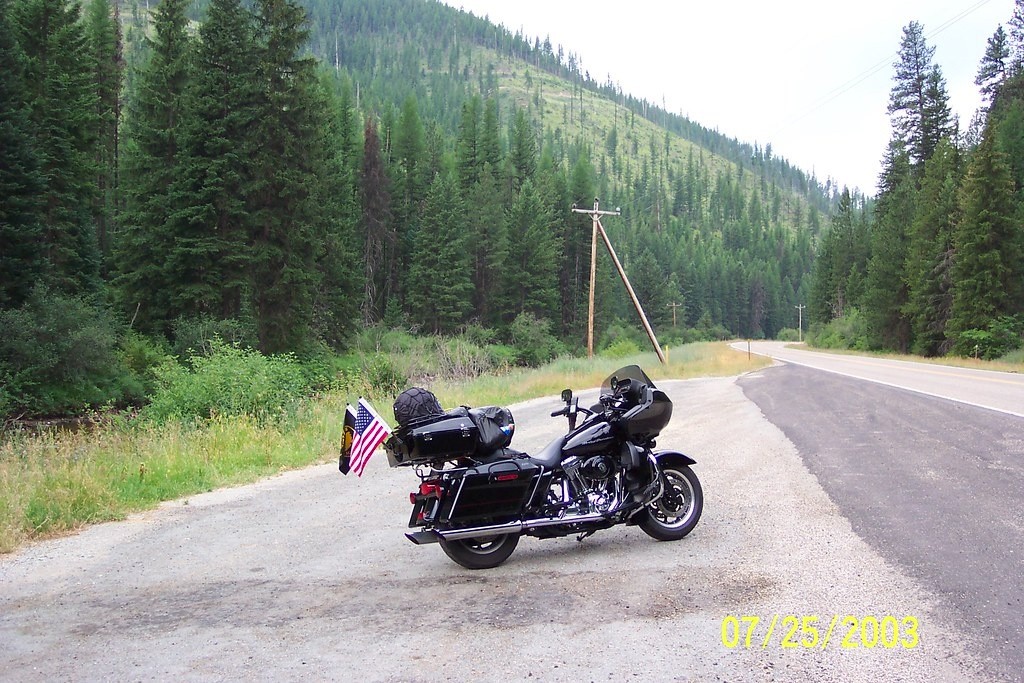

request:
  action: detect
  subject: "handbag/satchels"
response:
[467,406,514,452]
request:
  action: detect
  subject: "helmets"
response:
[394,387,445,427]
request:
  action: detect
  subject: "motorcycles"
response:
[385,365,703,570]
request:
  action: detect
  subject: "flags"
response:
[339,398,392,477]
